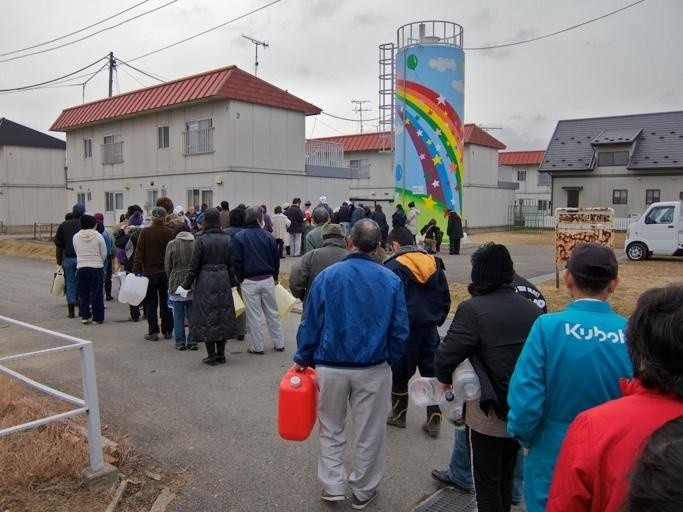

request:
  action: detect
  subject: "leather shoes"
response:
[216,354,226,362]
[203,355,218,366]
[431,470,475,494]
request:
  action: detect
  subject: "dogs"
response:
[436,231,444,252]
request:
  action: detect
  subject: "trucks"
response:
[623,199,683,262]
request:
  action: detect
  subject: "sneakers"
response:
[187,343,198,350]
[320,489,346,501]
[174,343,186,350]
[247,347,265,355]
[164,330,172,339]
[351,490,377,510]
[144,332,159,341]
[273,347,283,351]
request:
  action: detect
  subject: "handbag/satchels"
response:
[50,269,66,297]
[419,232,425,244]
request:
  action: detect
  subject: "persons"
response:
[545,283,682,510]
[618,415,682,511]
[271,195,463,259]
[507,243,636,511]
[292,219,409,510]
[52,196,285,368]
[383,225,450,438]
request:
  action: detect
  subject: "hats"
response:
[128,211,142,226]
[471,243,513,284]
[283,203,289,209]
[565,243,618,282]
[320,222,346,240]
[126,205,143,218]
[319,196,328,205]
[157,197,174,213]
[196,212,205,225]
[152,207,168,218]
[65,202,104,234]
[408,201,415,208]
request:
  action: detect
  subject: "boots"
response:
[386,393,409,428]
[421,407,442,437]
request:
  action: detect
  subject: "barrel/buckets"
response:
[49,267,66,296]
[231,286,246,318]
[278,359,318,440]
[118,273,149,306]
[405,359,481,428]
[275,283,296,318]
